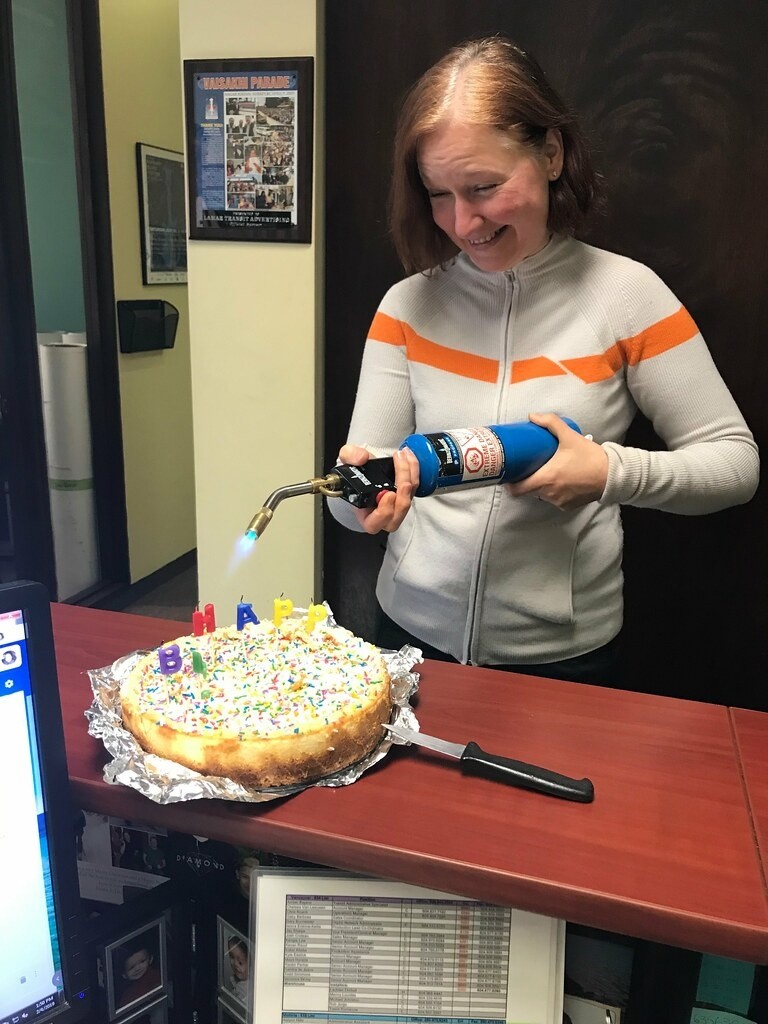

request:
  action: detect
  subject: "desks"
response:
[49,600,768,1018]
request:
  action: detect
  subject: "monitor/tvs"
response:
[0,580,97,1024]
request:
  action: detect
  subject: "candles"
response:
[234,595,262,630]
[192,600,217,636]
[192,651,208,678]
[272,592,293,628]
[306,597,330,634]
[158,637,182,676]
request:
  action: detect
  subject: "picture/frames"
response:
[186,57,312,242]
[104,913,168,1022]
[215,913,253,1015]
[135,141,188,286]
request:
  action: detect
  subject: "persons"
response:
[112,830,168,874]
[324,35,761,685]
[228,937,250,1004]
[117,944,161,1007]
[227,98,294,212]
[234,859,261,921]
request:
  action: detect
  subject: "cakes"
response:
[122,594,392,786]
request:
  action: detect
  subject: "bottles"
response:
[398,417,584,498]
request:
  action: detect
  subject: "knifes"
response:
[380,722,595,804]
